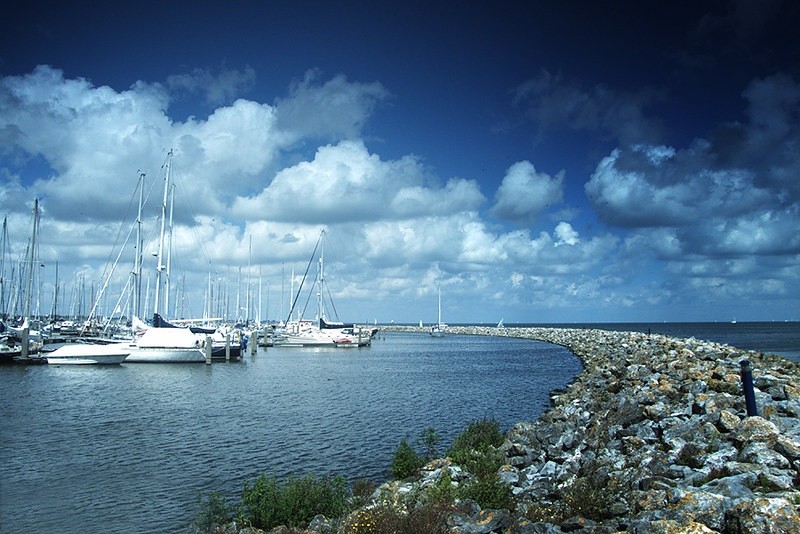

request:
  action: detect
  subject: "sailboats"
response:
[0,145,378,366]
[428,284,448,337]
